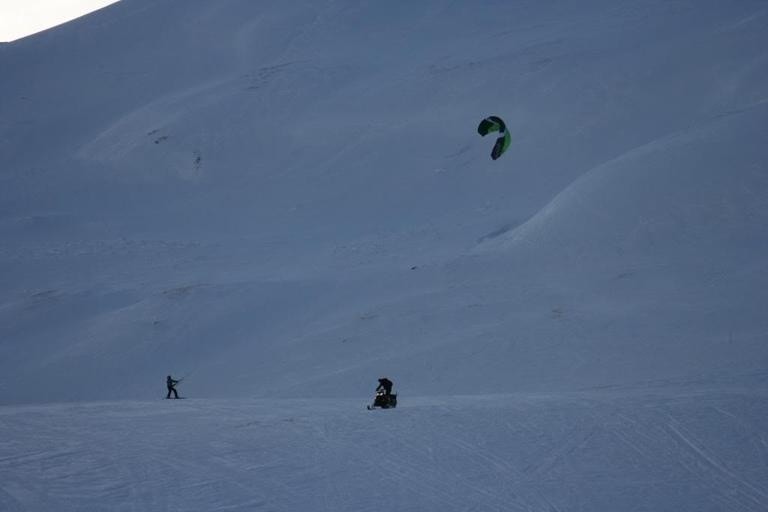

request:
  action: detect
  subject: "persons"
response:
[376,378,394,403]
[166,375,180,398]
